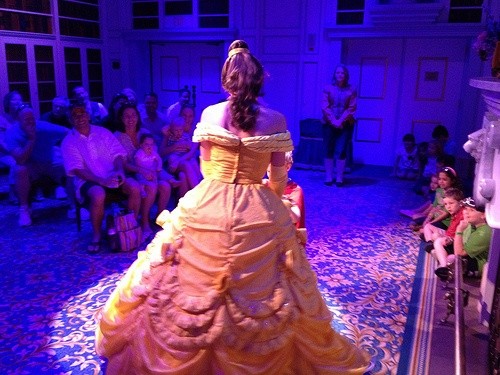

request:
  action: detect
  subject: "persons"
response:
[0,92,44,203]
[259,148,306,249]
[8,104,90,227]
[167,118,197,171]
[322,65,356,186]
[389,125,456,176]
[168,89,190,121]
[135,134,182,192]
[42,97,69,199]
[432,198,491,281]
[70,87,108,127]
[115,105,170,235]
[94,40,371,375]
[136,90,166,155]
[102,93,128,134]
[120,88,139,104]
[161,105,198,197]
[412,168,461,238]
[63,102,142,252]
[425,188,466,260]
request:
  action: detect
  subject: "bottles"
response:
[105,215,120,252]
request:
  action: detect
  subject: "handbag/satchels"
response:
[106,209,144,252]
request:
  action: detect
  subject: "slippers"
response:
[87,238,102,252]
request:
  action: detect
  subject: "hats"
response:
[460,197,485,212]
[440,166,456,183]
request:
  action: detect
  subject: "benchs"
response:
[72,193,129,233]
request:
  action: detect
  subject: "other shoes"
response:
[435,267,449,283]
[19,205,33,229]
[324,177,343,188]
[425,240,433,246]
[423,245,434,254]
